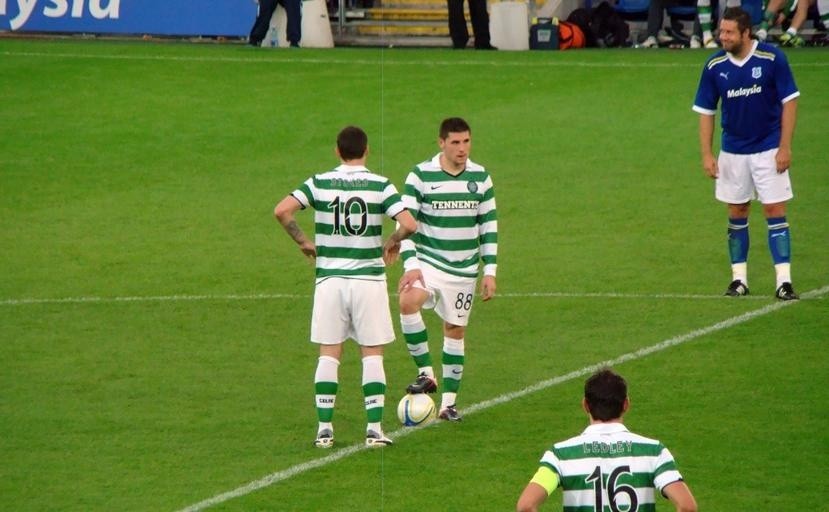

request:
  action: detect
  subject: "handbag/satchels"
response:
[530,17,585,50]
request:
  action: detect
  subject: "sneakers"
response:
[641,36,658,48]
[439,405,461,421]
[366,435,392,447]
[315,434,333,448]
[776,283,798,300]
[407,372,436,393]
[690,35,719,49]
[725,280,749,295]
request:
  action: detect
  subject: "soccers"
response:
[397,393,437,428]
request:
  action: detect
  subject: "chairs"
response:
[611,0,774,47]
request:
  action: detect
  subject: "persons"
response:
[692,7,800,302]
[274,126,417,450]
[447,0,499,50]
[515,364,699,512]
[249,0,301,48]
[603,0,829,48]
[395,118,497,422]
